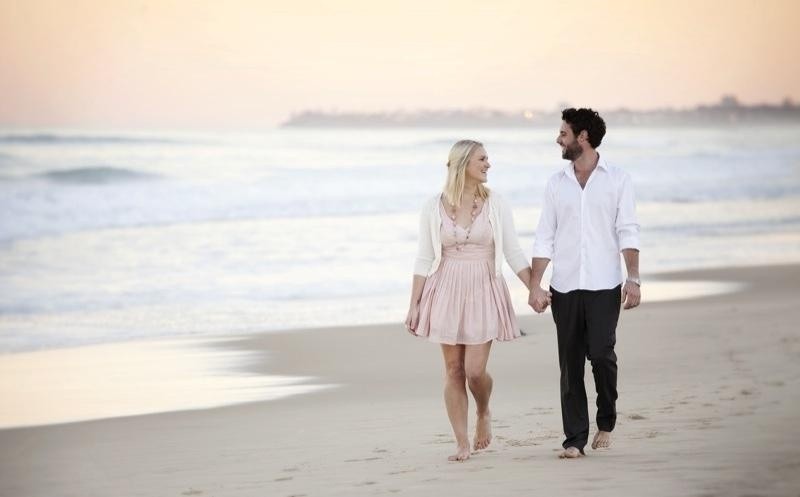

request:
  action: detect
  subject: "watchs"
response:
[626,277,642,286]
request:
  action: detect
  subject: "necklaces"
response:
[448,187,479,251]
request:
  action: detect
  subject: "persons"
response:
[528,109,642,458]
[404,141,550,461]
